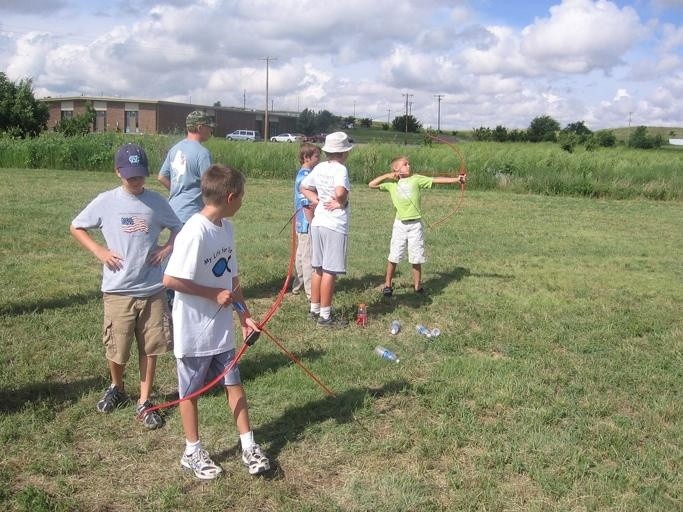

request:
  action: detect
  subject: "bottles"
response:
[391,320,400,335]
[416,325,432,340]
[357,304,366,328]
[431,329,442,338]
[375,346,400,365]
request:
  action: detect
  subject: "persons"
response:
[69,144,182,429]
[292,142,321,305]
[298,130,356,330]
[159,109,212,227]
[163,164,272,481]
[367,155,467,298]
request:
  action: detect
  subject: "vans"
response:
[224,128,260,143]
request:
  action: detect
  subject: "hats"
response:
[186,111,218,128]
[116,143,149,179]
[321,132,353,153]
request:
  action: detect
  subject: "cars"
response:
[270,131,306,144]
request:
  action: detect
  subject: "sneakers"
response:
[382,286,394,297]
[415,287,425,294]
[134,398,162,429]
[180,449,223,480]
[316,315,350,330]
[97,384,125,413]
[292,288,302,295]
[306,309,318,321]
[242,444,270,474]
